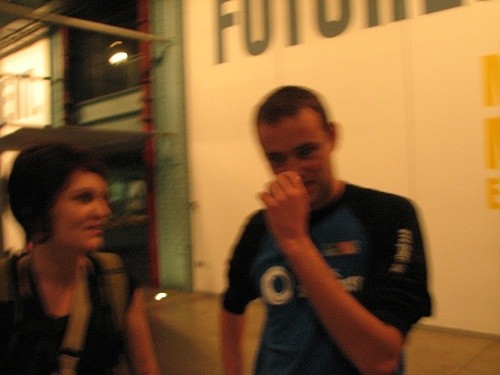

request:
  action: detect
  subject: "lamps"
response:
[107,39,128,65]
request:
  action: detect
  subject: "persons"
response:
[218,85,432,375]
[0,143,162,375]
